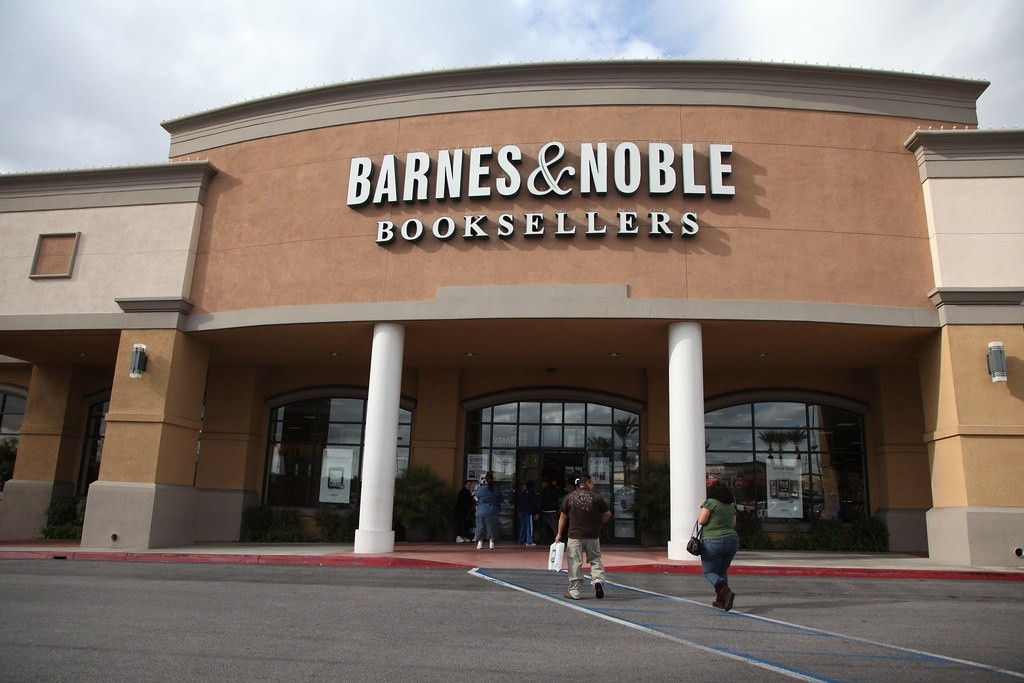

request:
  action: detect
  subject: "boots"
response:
[712,581,735,612]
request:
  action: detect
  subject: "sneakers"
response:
[455,535,495,549]
[525,543,536,547]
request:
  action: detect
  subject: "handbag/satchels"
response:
[547,540,565,573]
[686,520,704,556]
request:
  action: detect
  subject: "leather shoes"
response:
[563,591,580,599]
[594,582,604,599]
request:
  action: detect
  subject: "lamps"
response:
[987,339,1010,384]
[127,342,148,379]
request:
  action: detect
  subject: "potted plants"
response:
[629,462,669,546]
[392,461,449,541]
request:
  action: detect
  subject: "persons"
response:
[475,473,501,549]
[518,480,538,547]
[555,474,612,600]
[550,476,574,497]
[698,481,740,612]
[456,480,474,543]
[540,478,558,544]
